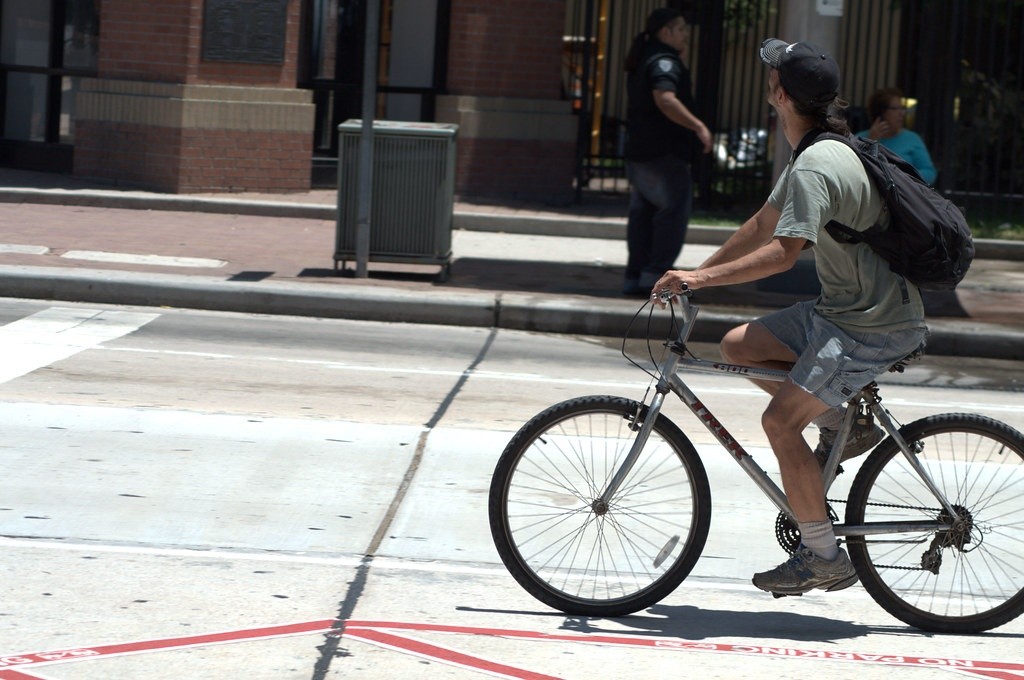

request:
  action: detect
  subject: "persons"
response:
[648,36,927,597]
[855,89,937,188]
[623,7,714,296]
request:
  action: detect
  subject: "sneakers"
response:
[752,544,859,594]
[811,414,886,467]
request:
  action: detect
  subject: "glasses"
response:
[888,106,908,113]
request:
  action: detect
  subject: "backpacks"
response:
[791,128,975,294]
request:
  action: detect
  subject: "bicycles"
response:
[487,279,1024,635]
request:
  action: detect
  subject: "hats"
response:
[758,36,841,108]
